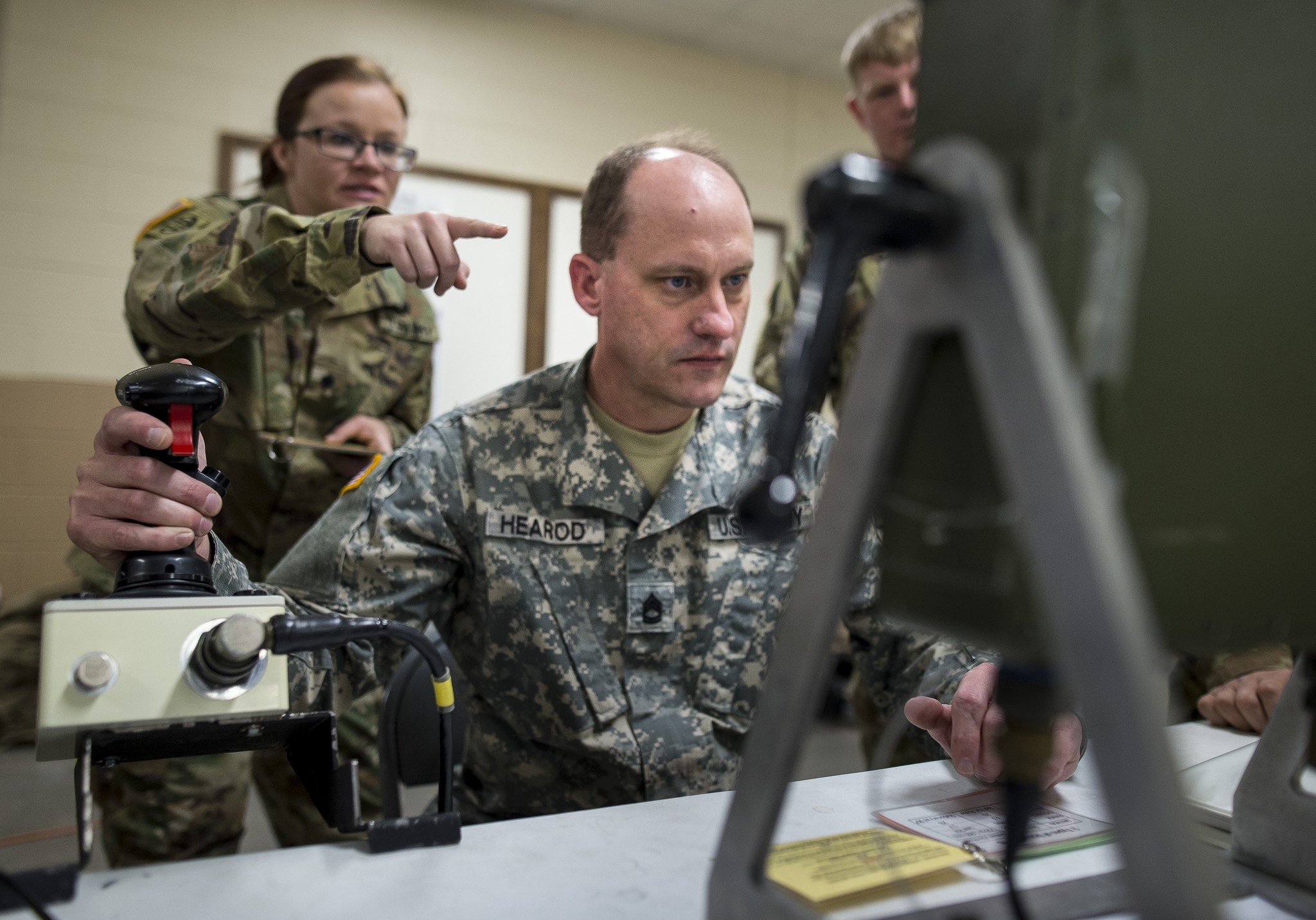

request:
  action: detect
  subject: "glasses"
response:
[294,127,417,173]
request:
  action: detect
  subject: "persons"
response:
[1163,650,1297,742]
[65,123,1095,830]
[83,52,515,859]
[750,2,926,428]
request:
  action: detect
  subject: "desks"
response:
[6,718,1314,920]
